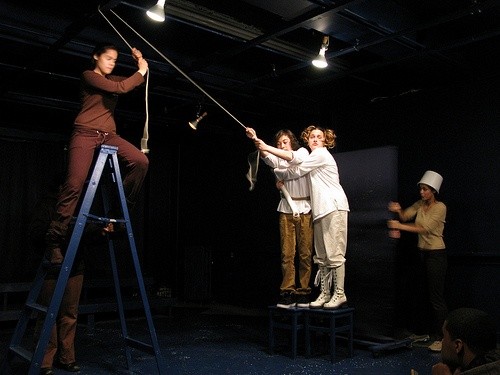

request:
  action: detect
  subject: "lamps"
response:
[311,35,330,68]
[188,103,208,130]
[146,0,166,22]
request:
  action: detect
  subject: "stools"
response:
[303,307,356,363]
[266,305,304,360]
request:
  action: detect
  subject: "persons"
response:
[43,45,150,265]
[245,124,349,311]
[430,309,500,375]
[35,176,117,375]
[385,170,449,352]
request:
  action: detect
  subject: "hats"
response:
[416,171,444,196]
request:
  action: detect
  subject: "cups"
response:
[388,221,400,238]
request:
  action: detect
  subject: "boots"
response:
[310,266,333,309]
[323,264,347,310]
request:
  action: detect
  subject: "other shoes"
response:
[44,247,65,265]
[428,341,444,352]
[40,368,55,375]
[297,295,311,307]
[58,362,82,374]
[276,294,296,308]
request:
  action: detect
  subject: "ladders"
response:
[4,144,166,375]
